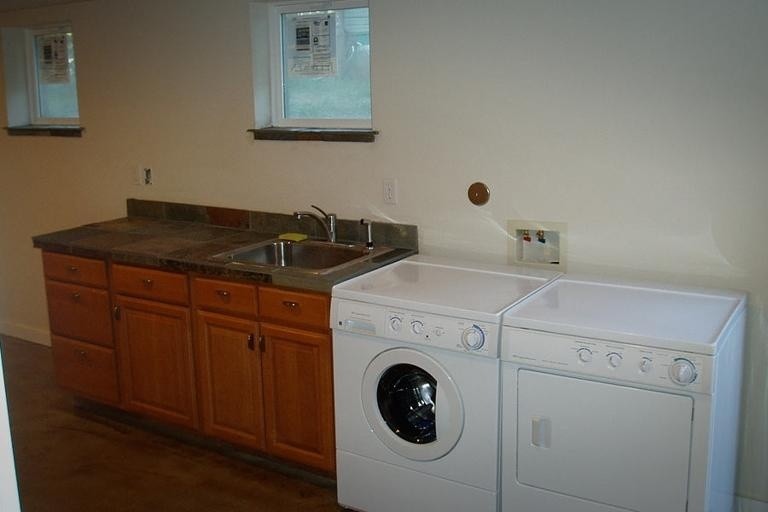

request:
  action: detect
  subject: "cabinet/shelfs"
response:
[189,277,336,476]
[41,250,198,435]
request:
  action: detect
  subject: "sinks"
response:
[222,232,373,273]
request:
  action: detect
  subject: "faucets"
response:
[294,202,338,243]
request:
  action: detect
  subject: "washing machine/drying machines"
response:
[324,250,564,512]
[496,276,747,512]
